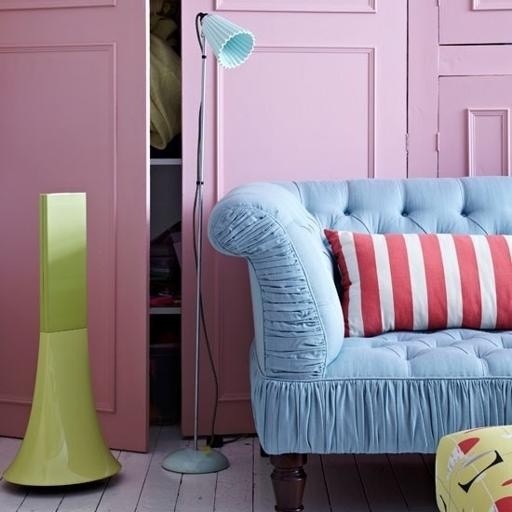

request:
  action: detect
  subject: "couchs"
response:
[209,178,512,511]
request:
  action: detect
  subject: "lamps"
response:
[160,11,256,476]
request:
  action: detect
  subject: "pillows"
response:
[325,228,512,336]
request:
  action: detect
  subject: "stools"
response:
[435,425,512,512]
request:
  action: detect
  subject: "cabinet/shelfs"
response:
[407,0,511,178]
[0,0,408,453]
